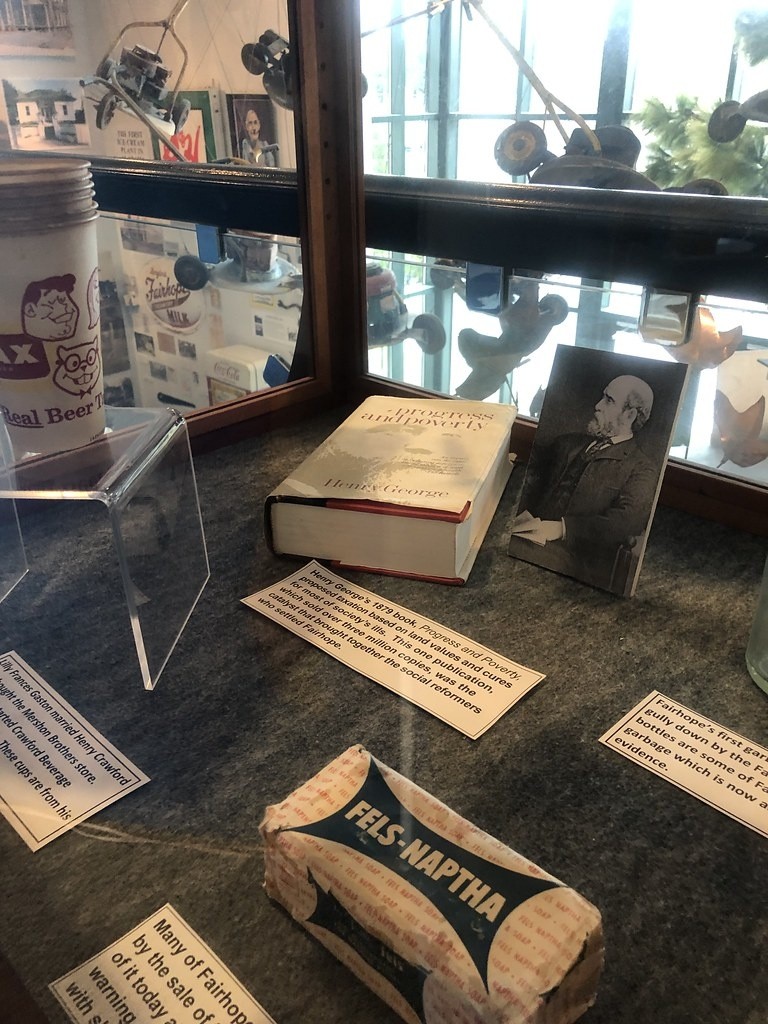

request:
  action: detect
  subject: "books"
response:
[262,393,518,588]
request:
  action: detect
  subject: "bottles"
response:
[364,262,409,342]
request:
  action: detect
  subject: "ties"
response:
[536,437,613,520]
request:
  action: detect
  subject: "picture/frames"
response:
[219,90,299,169]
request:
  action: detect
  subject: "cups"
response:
[745,557,768,694]
[0,156,107,453]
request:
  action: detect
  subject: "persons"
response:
[508,376,657,596]
